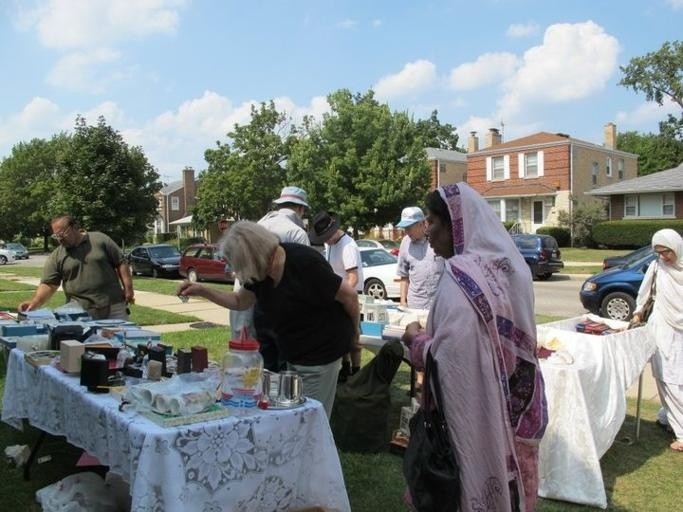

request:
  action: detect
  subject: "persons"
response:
[401,180,548,512]
[176,220,360,422]
[18,215,134,321]
[396,206,447,385]
[230,187,313,341]
[632,228,683,452]
[310,211,364,377]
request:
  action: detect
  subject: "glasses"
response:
[50,225,71,241]
[653,248,670,258]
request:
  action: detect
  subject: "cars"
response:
[579,253,657,320]
[358,247,401,298]
[601,244,651,270]
[355,238,398,260]
[122,245,181,278]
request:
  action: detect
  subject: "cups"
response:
[246,368,272,401]
[141,390,186,413]
[276,371,306,407]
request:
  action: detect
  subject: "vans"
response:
[0,240,15,265]
[509,234,563,279]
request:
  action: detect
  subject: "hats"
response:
[395,206,425,228]
[272,186,308,207]
[310,210,341,245]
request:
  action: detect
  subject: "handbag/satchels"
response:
[641,296,654,321]
[403,406,460,512]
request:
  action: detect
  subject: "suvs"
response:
[178,243,235,282]
[4,243,28,259]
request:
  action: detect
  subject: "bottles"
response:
[222,325,264,418]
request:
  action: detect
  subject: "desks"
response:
[1,341,351,512]
[535,313,658,509]
[356,300,431,400]
